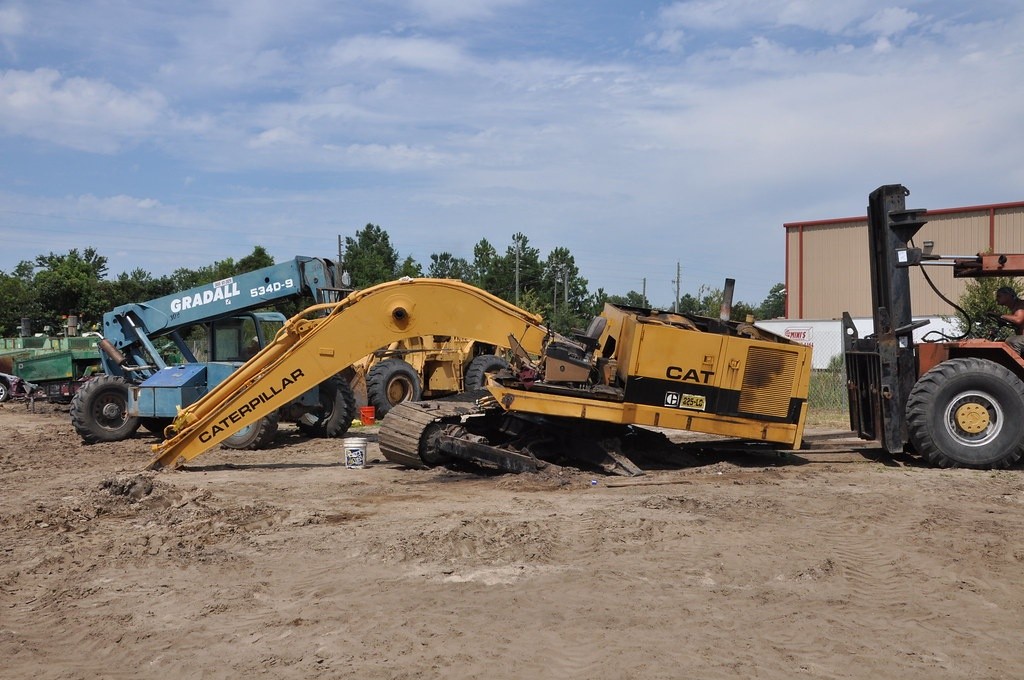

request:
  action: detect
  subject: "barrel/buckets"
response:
[361,406,376,425]
[343,438,368,470]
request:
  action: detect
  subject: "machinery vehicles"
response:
[354,333,477,431]
[69,234,420,453]
[142,277,813,483]
[0,315,106,403]
[802,182,1024,471]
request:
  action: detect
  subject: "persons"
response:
[986,287,1024,355]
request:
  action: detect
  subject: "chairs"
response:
[549,315,607,362]
